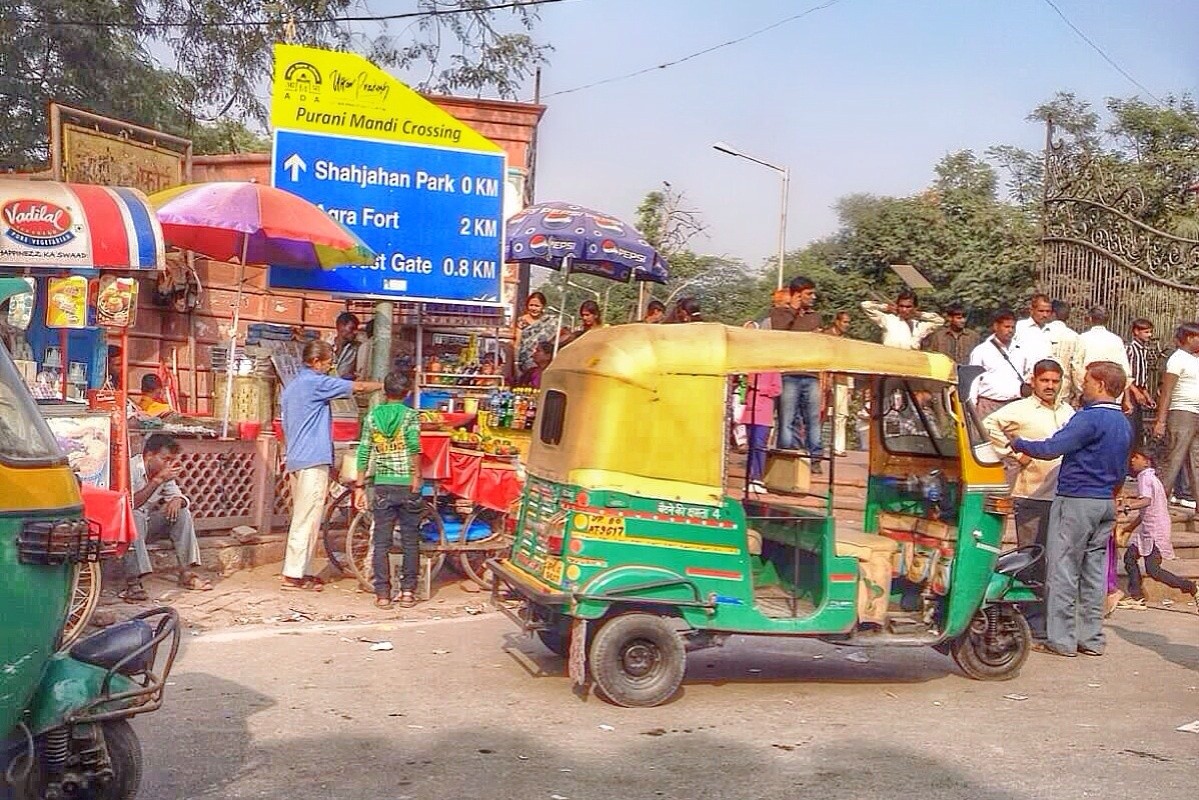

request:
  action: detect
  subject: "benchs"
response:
[742,495,896,566]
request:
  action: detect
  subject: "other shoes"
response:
[1030,627,1047,639]
[1170,496,1180,506]
[811,461,823,474]
[834,451,846,457]
[1180,499,1196,510]
[1115,597,1147,611]
[737,444,749,454]
[1107,588,1124,613]
[742,479,768,494]
[1194,583,1199,613]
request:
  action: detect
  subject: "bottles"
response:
[427,355,540,431]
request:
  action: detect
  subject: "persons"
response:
[970,294,1199,657]
[106,343,181,417]
[732,277,982,495]
[125,433,214,604]
[514,292,703,389]
[484,352,502,365]
[279,312,424,610]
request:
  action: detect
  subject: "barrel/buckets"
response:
[238,422,262,442]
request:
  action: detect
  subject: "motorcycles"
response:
[1,312,181,800]
[482,324,1050,709]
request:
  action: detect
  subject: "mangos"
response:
[453,432,519,456]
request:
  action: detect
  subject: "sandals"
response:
[374,596,393,610]
[125,580,146,600]
[400,592,422,608]
[1032,642,1064,655]
[280,575,327,592]
[178,569,214,590]
[1077,644,1102,656]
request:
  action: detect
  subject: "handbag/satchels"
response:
[1021,382,1032,398]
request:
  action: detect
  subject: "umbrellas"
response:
[147,178,379,442]
[504,202,670,360]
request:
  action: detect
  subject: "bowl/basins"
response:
[451,441,478,450]
[419,421,447,431]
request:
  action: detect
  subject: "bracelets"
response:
[356,485,365,489]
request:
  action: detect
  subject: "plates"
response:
[99,288,131,320]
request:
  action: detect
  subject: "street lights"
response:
[708,143,795,296]
